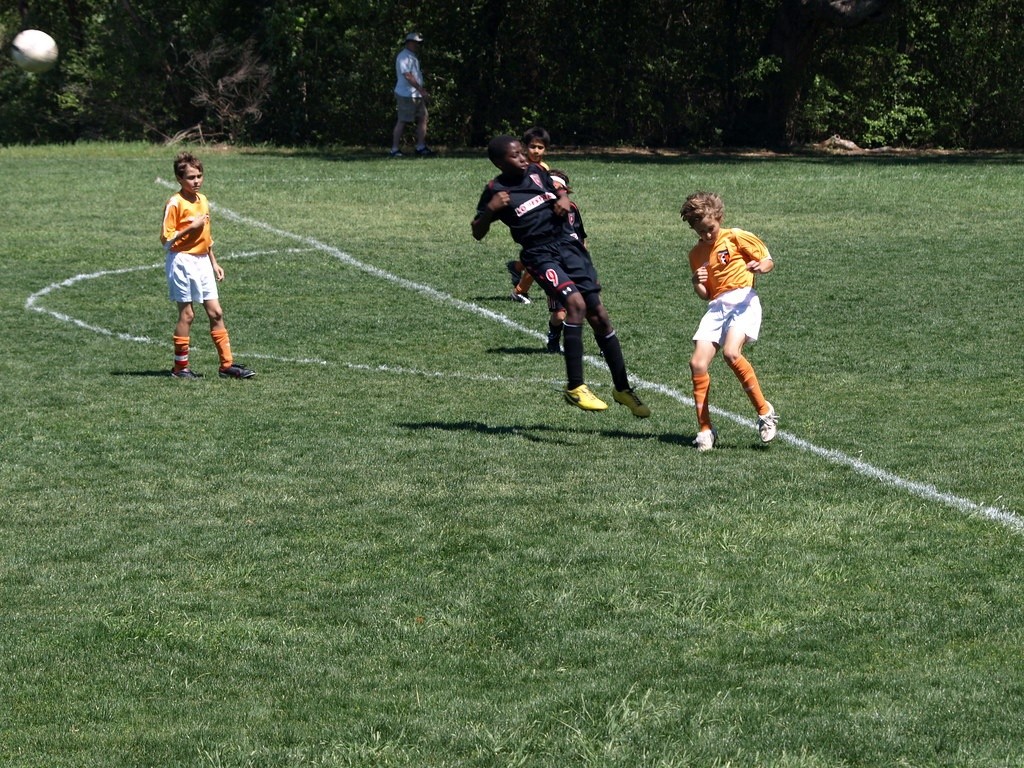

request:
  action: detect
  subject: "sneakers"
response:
[170,369,205,381]
[759,400,777,442]
[612,388,651,417]
[563,383,609,410]
[219,364,255,378]
[695,426,718,452]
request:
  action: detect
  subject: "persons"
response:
[471,125,652,419]
[159,150,255,381]
[388,32,436,159]
[679,191,780,454]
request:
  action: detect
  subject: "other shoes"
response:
[413,144,433,159]
[507,260,523,287]
[547,333,561,352]
[390,148,409,159]
[507,290,535,302]
[600,350,604,357]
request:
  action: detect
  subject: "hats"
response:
[405,33,424,42]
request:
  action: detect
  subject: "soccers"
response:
[11,29,59,73]
